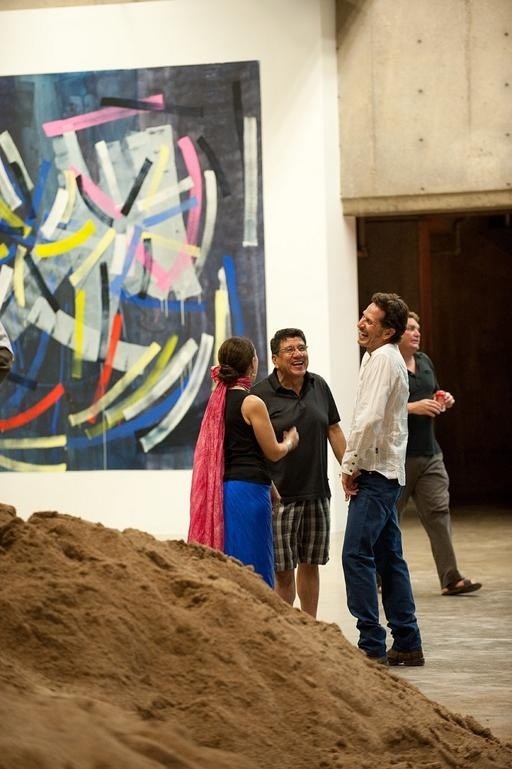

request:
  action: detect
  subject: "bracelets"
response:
[285,439,295,453]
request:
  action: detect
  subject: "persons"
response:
[375,310,481,596]
[189,337,300,593]
[250,327,346,620]
[333,294,425,669]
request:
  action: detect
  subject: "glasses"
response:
[276,344,308,353]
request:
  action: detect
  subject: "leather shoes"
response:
[367,647,424,666]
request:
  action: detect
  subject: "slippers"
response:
[441,578,482,595]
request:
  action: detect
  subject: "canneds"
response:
[436,390,446,413]
